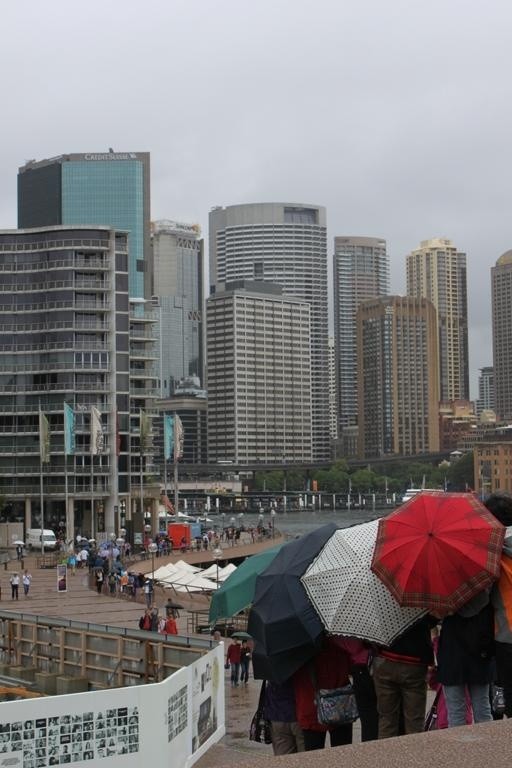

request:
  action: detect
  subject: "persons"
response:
[139,598,185,633]
[181,530,219,553]
[22,570,32,596]
[75,543,155,607]
[10,570,20,601]
[225,487,511,767]
[155,534,175,557]
[56,530,65,564]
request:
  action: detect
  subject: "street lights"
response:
[221,512,243,548]
[259,508,276,540]
[184,521,189,550]
[109,528,126,572]
[211,548,223,590]
[144,524,158,609]
[204,512,208,530]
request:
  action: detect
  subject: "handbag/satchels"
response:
[249,679,273,744]
[423,687,442,731]
[307,659,361,726]
[492,686,506,714]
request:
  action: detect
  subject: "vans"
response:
[27,529,57,551]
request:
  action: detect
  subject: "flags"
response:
[139,410,153,458]
[90,404,103,455]
[40,412,51,461]
[174,413,184,457]
[164,414,174,460]
[64,405,76,452]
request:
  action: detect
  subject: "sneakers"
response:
[231,678,249,685]
